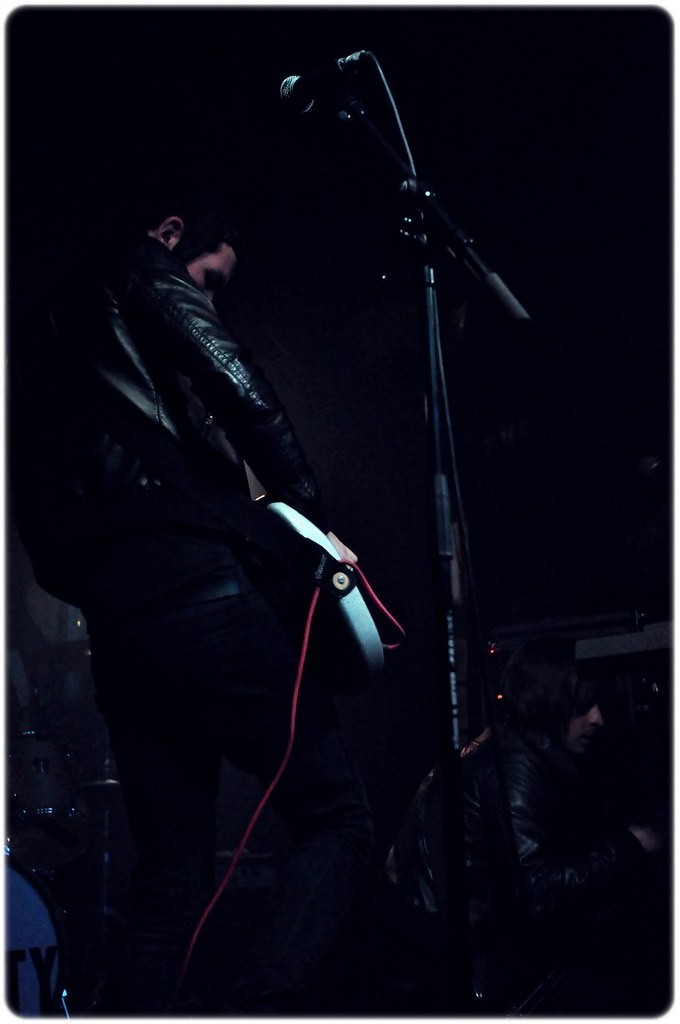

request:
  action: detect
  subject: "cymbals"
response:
[72,778,122,786]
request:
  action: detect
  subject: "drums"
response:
[9,725,89,865]
[9,857,76,1013]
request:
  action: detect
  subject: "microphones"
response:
[28,681,49,775]
[279,50,368,114]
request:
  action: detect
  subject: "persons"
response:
[10,204,376,1014]
[386,635,667,1014]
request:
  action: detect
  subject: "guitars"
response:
[253,499,412,675]
